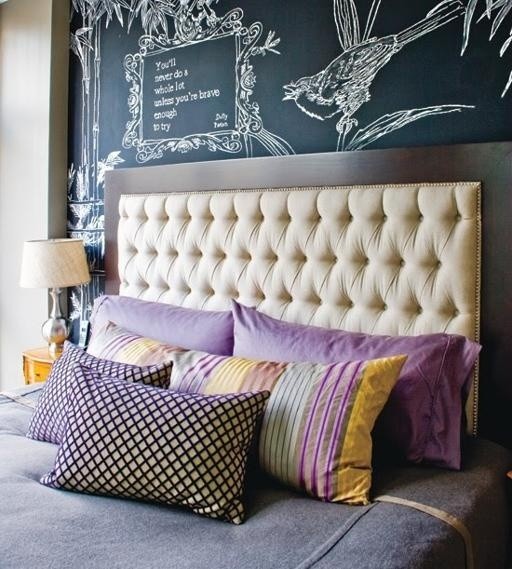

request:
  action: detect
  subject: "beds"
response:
[0,140,511,569]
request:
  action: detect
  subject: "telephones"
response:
[79,320,90,347]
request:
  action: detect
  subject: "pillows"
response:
[167,350,408,508]
[90,293,235,357]
[25,341,174,448]
[39,363,272,525]
[423,337,482,469]
[230,298,452,466]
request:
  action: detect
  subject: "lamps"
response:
[19,238,92,360]
